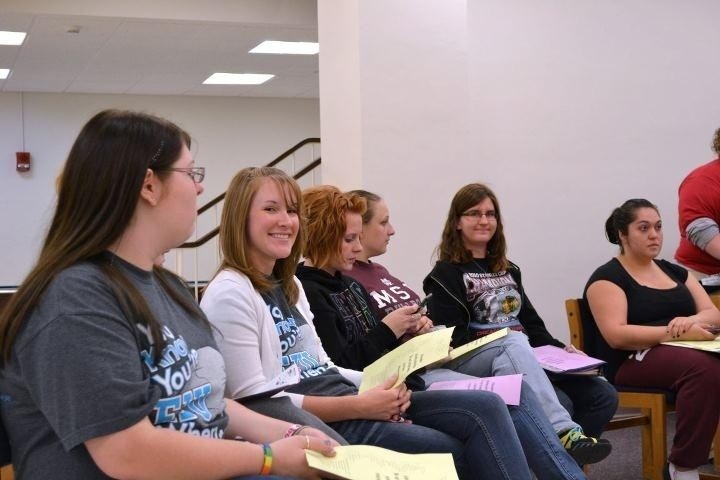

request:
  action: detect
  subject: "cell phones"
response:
[413,293,432,313]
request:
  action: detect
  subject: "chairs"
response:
[566,296,720,480]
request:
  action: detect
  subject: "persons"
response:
[672,128,720,291]
[580,197,720,480]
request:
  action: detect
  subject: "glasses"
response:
[150,165,206,184]
[460,209,499,222]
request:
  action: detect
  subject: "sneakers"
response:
[668,462,701,480]
[562,430,612,464]
